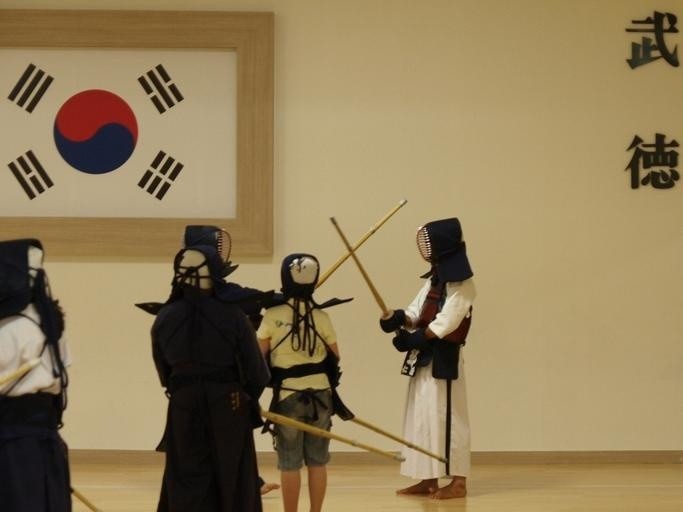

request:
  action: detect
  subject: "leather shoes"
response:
[378,308,407,334]
[391,328,428,353]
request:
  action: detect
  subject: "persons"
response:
[253,251,342,511]
[377,214,477,503]
[183,218,292,493]
[0,237,73,512]
[148,246,270,511]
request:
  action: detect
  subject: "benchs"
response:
[0,48,236,219]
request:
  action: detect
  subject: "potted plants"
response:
[0,6,278,270]
